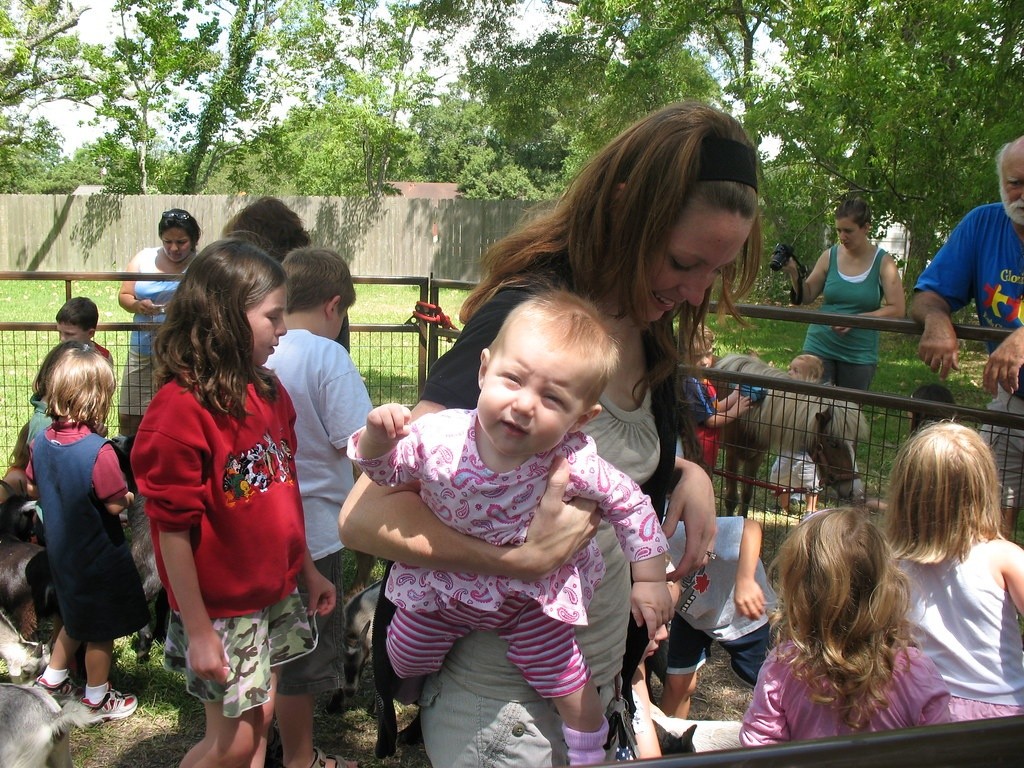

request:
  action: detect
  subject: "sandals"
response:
[266,726,362,768]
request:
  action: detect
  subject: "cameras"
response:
[153,305,166,315]
[769,243,795,271]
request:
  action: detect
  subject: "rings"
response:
[705,550,717,560]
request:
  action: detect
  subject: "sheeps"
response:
[342,575,423,759]
[649,701,743,756]
[0,433,173,768]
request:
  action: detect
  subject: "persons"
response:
[0,197,373,768]
[769,196,906,389]
[338,104,763,768]
[911,135,1024,539]
[660,320,1024,748]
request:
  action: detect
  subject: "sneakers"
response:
[77,688,139,724]
[32,675,86,706]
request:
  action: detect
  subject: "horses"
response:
[706,352,869,518]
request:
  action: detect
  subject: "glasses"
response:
[162,212,191,224]
